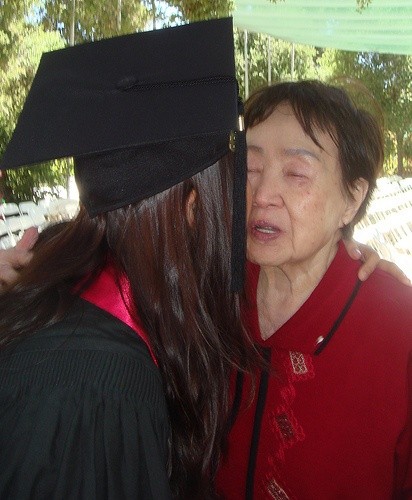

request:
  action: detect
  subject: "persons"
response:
[0,80,411,499]
[0,18,270,500]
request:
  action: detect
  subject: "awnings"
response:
[232,1,412,56]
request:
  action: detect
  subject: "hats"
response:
[0,15,246,219]
